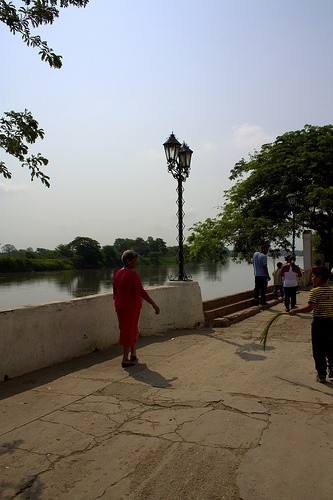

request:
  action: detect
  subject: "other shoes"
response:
[316,374,326,383]
[122,361,135,368]
[328,374,333,381]
[131,356,136,361]
[292,306,298,309]
[285,308,289,312]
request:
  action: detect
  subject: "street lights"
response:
[162,130,193,281]
[285,190,303,256]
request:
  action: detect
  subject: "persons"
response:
[252,245,271,306]
[287,266,333,383]
[111,249,160,365]
[272,255,333,313]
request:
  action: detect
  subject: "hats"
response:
[121,250,138,262]
